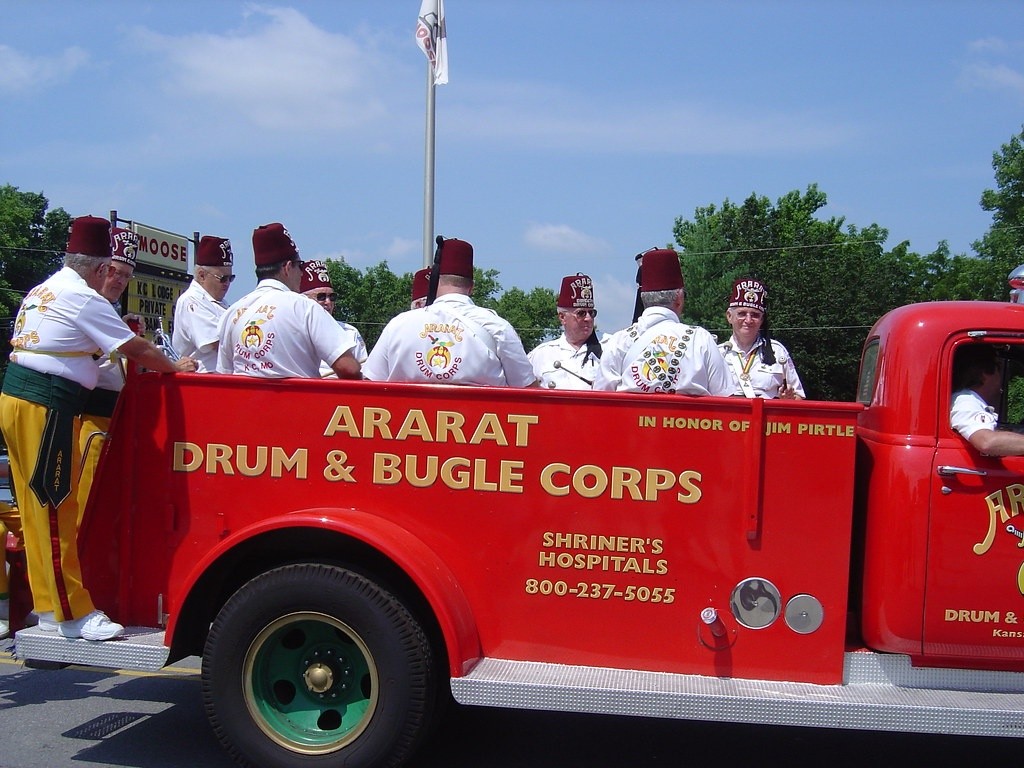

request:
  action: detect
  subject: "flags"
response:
[415,0,448,86]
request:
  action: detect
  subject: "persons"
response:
[411,269,431,309]
[719,279,806,400]
[216,222,361,378]
[949,346,1024,456]
[360,238,537,388]
[593,250,728,395]
[299,261,368,379]
[0,215,197,640]
[172,236,235,373]
[528,274,603,390]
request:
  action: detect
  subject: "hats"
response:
[632,247,684,324]
[729,278,776,367]
[253,223,299,264]
[67,215,114,257]
[300,260,333,294]
[196,236,234,266]
[558,272,594,308]
[426,236,473,305]
[111,227,139,268]
[412,266,432,301]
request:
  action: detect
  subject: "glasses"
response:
[107,264,116,278]
[565,309,597,319]
[308,292,337,301]
[293,261,306,270]
[732,311,764,320]
[204,270,236,284]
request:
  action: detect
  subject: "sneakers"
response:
[58,609,124,641]
[38,611,58,629]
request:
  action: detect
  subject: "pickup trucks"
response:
[15,263,1024,768]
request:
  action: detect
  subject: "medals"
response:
[740,374,750,380]
